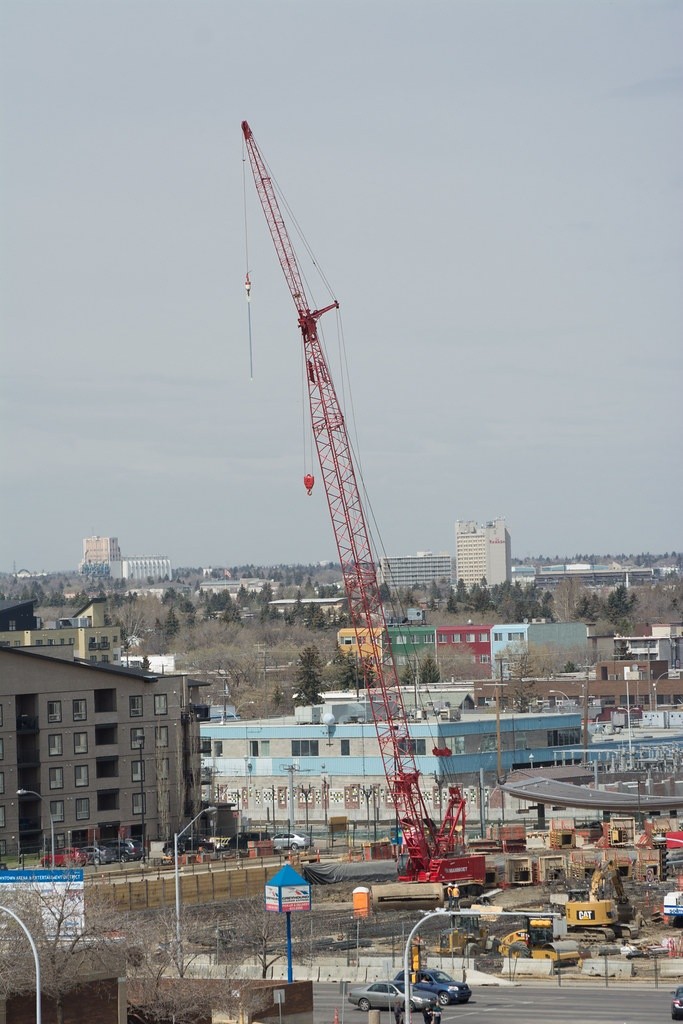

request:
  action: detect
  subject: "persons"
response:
[420,976,428,983]
[432,1000,442,1024]
[423,1007,432,1024]
[393,1002,400,1024]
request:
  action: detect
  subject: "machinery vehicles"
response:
[242,121,486,895]
[498,916,583,968]
[564,859,643,944]
[431,914,501,958]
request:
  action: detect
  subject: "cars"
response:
[228,831,270,849]
[40,847,88,867]
[162,835,215,857]
[394,968,472,1006]
[270,833,314,851]
[348,980,438,1013]
[82,845,116,865]
[670,986,683,1020]
[103,839,143,862]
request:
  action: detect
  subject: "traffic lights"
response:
[660,850,669,881]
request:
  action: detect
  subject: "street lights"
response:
[218,710,237,718]
[618,707,639,770]
[136,735,145,864]
[17,791,55,869]
[174,807,217,961]
[320,771,328,827]
[654,672,676,712]
[529,754,534,769]
[550,690,572,713]
[364,789,372,840]
[234,701,254,719]
